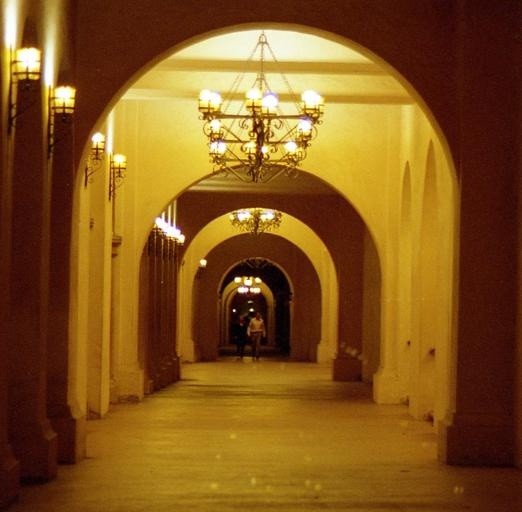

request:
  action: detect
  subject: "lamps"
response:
[83,133,126,202]
[196,27,327,239]
[7,38,77,161]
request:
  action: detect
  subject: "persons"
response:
[246,311,266,363]
[230,313,250,362]
